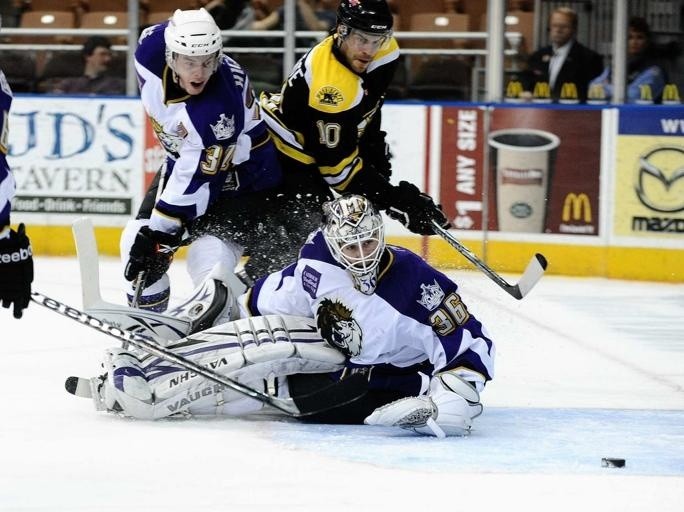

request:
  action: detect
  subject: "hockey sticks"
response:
[28,290,368,417]
[427,216,547,300]
[64,161,168,397]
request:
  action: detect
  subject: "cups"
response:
[488,130,560,233]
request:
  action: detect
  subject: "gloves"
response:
[370,131,391,181]
[384,181,451,235]
[0,222,33,318]
[124,225,185,290]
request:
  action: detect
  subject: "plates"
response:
[503,98,525,104]
[587,100,607,106]
[635,99,654,106]
[558,98,580,106]
[533,98,554,104]
[662,100,681,106]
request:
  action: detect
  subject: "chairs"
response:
[0,0,537,100]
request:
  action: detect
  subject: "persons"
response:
[515,6,604,105]
[48,34,126,96]
[0,67,33,320]
[585,16,670,103]
[122,7,287,316]
[244,0,452,285]
[91,193,497,440]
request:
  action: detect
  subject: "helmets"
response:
[164,6,223,75]
[323,193,385,296]
[335,1,392,53]
